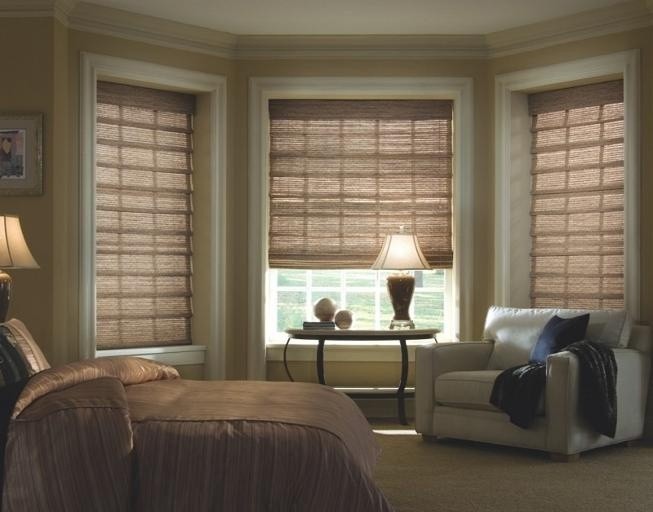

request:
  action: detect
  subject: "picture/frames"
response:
[0,110,44,198]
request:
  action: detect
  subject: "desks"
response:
[284,328,439,427]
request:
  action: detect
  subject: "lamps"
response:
[0,212,42,322]
[370,234,432,330]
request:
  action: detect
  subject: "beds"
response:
[3,375,373,512]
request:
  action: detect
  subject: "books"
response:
[302,320,336,332]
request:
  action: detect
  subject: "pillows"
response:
[528,313,591,364]
[0,317,52,392]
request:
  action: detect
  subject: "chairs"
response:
[414,304,653,464]
